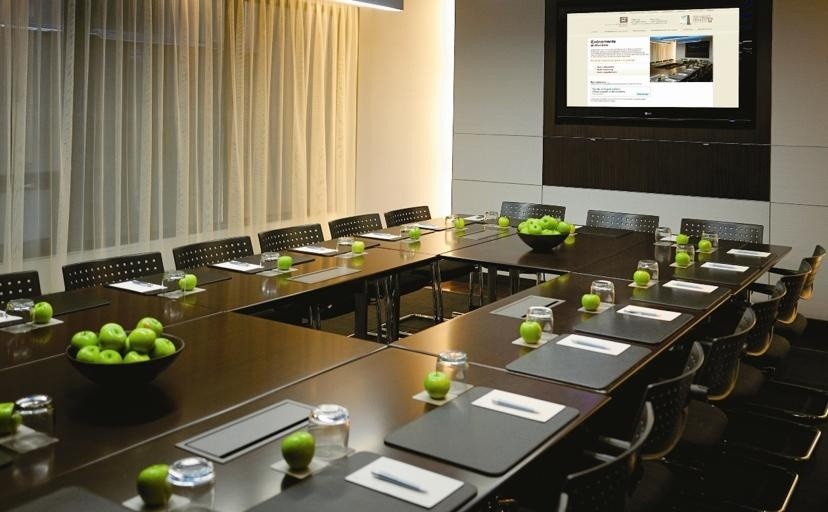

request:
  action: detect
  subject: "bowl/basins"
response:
[65,332,184,382]
[515,232,570,251]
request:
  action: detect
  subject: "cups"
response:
[310,404,351,457]
[169,457,215,512]
[10,452,56,498]
[484,211,497,223]
[6,298,35,331]
[399,242,416,259]
[654,227,671,244]
[445,232,459,245]
[260,252,280,274]
[336,237,354,258]
[436,351,467,393]
[702,234,718,249]
[590,279,615,305]
[161,298,185,319]
[526,305,553,338]
[638,260,659,284]
[260,276,281,296]
[161,270,185,298]
[445,216,457,231]
[399,225,416,243]
[675,244,695,263]
[336,258,353,273]
[10,394,54,451]
[7,333,34,360]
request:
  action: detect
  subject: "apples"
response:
[698,240,712,251]
[633,270,650,285]
[677,234,689,245]
[277,256,292,270]
[498,217,509,226]
[35,302,54,323]
[698,252,711,263]
[518,216,574,234]
[455,219,465,228]
[519,322,542,343]
[498,229,508,238]
[546,273,572,287]
[580,312,592,320]
[70,317,176,363]
[633,288,651,299]
[454,230,465,236]
[582,294,600,310]
[24,329,53,344]
[282,432,314,469]
[410,241,420,250]
[564,236,575,245]
[423,372,451,398]
[675,253,690,265]
[278,273,291,284]
[0,402,23,433]
[351,241,364,252]
[674,266,694,278]
[179,293,197,305]
[409,228,422,238]
[518,347,534,358]
[137,464,171,505]
[179,274,197,291]
[352,255,364,265]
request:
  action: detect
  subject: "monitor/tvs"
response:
[556,0,756,128]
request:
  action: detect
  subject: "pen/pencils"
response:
[708,263,737,269]
[570,337,612,350]
[671,281,703,289]
[624,310,661,316]
[735,250,760,255]
[491,399,540,413]
[370,469,428,493]
